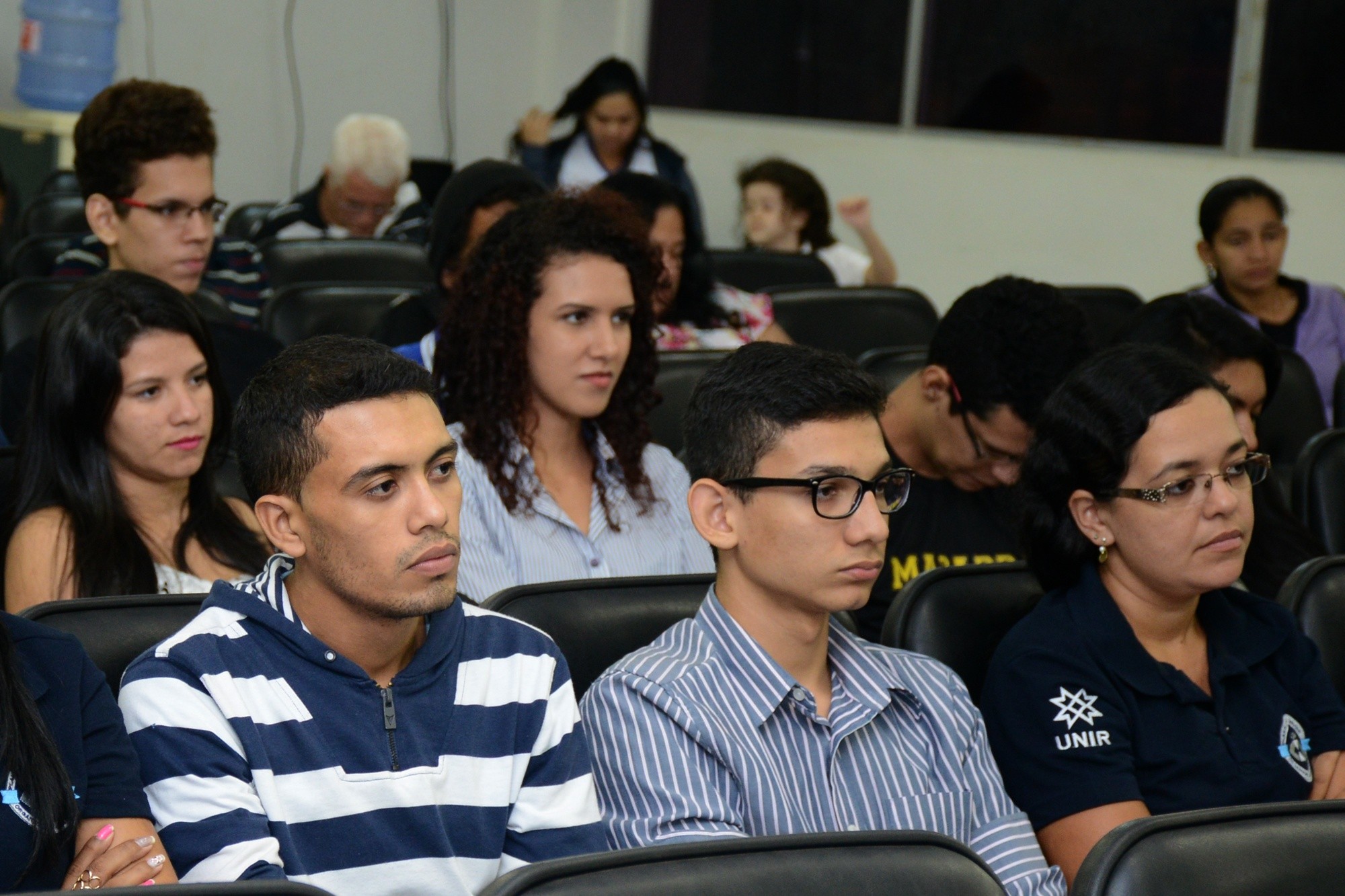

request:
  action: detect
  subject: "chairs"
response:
[0,163,1344,896]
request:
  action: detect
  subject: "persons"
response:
[0,48,1342,894]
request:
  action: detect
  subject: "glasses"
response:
[950,385,1048,464]
[1094,452,1270,505]
[723,468,917,520]
[117,198,228,221]
